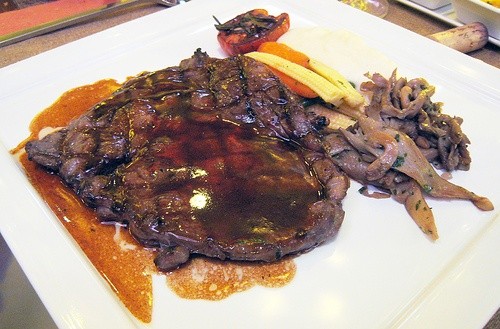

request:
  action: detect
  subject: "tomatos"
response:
[213,10,290,55]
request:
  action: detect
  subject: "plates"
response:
[0,0,500,329]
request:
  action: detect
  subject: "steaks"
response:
[25,48,350,273]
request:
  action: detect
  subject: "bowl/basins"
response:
[410,0,452,10]
[452,0,500,40]
